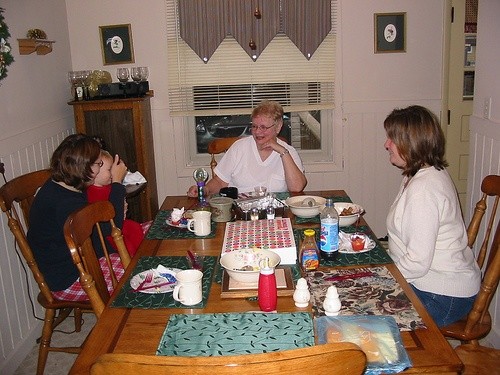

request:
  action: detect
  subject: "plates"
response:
[338,238,376,254]
[165,219,193,228]
[228,268,288,291]
[129,267,183,294]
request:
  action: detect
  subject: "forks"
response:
[134,273,153,292]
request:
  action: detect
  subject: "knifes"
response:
[128,281,175,293]
[324,272,373,281]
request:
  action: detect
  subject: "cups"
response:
[140,66,149,81]
[172,268,204,307]
[209,197,233,222]
[187,211,211,236]
[254,185,267,198]
[220,186,238,199]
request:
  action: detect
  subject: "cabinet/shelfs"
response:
[444,0,478,194]
[67,91,158,225]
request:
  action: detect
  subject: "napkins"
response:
[134,264,178,291]
[171,206,185,222]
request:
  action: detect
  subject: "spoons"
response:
[186,249,202,271]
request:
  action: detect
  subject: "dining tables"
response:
[70,190,469,375]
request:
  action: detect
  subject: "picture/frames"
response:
[375,12,406,53]
[99,24,135,65]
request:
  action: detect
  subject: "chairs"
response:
[0,165,131,375]
[209,136,288,179]
[438,175,500,375]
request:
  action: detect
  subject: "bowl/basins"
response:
[333,202,364,227]
[220,247,281,283]
[285,195,327,218]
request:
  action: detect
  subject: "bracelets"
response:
[279,149,289,157]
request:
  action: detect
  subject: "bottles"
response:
[266,205,275,219]
[320,198,339,261]
[323,286,341,317]
[299,229,320,277]
[250,207,259,221]
[257,256,278,312]
[293,278,310,307]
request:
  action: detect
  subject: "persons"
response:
[186,99,308,197]
[85,149,146,259]
[26,133,154,302]
[383,104,482,328]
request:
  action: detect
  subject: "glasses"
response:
[251,124,276,131]
[93,160,103,167]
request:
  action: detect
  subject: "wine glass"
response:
[131,67,142,84]
[116,68,129,85]
[68,70,101,101]
